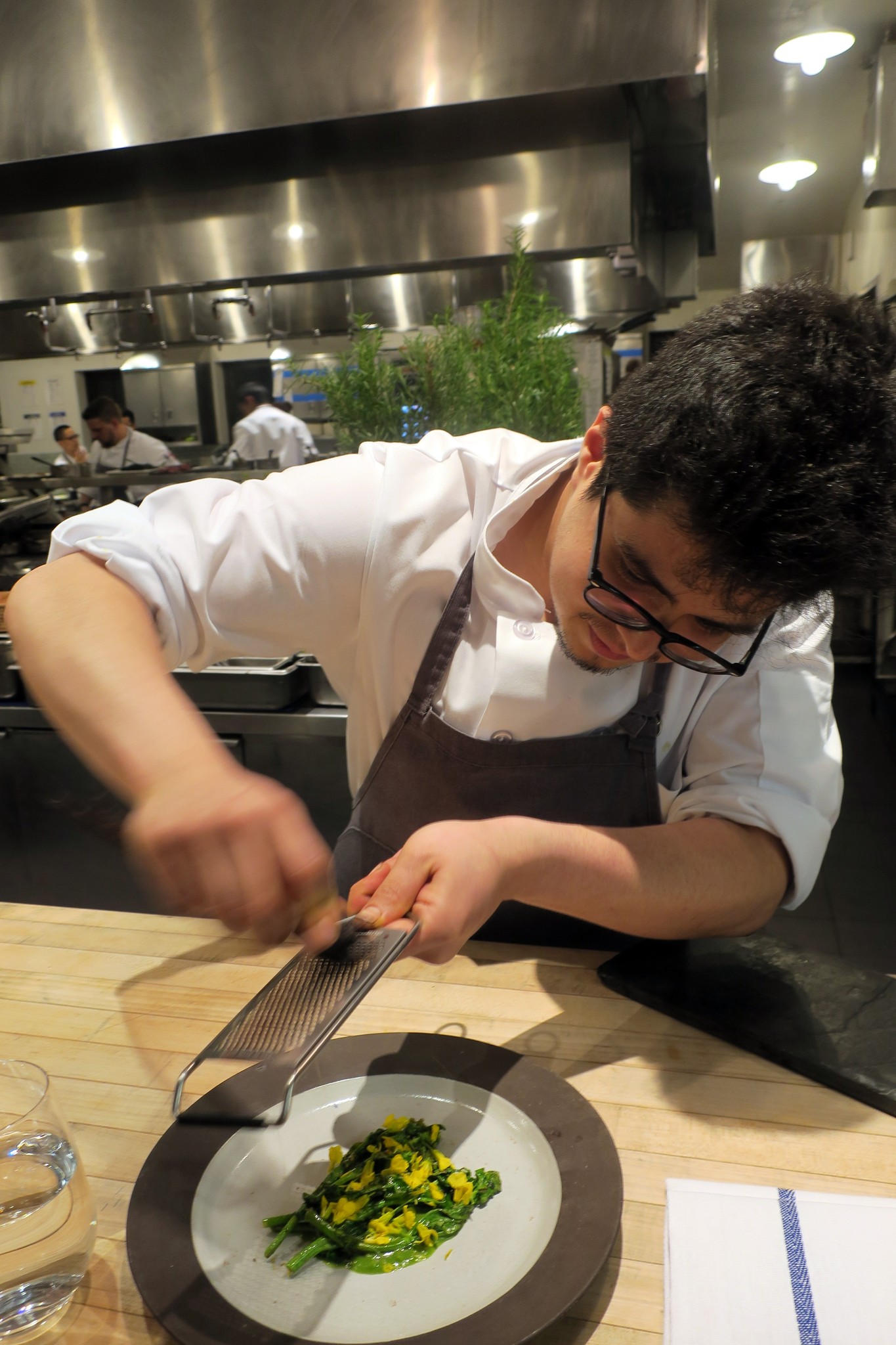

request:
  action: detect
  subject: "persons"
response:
[52,396,182,515]
[220,381,320,468]
[3,282,895,966]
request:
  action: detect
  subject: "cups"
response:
[0,1059,96,1345]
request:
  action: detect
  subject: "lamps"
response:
[758,145,816,191]
[772,3,855,78]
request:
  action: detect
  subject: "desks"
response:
[0,899,895,1342]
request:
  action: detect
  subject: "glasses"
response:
[584,464,778,676]
[60,433,78,440]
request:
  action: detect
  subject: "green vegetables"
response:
[267,1117,504,1279]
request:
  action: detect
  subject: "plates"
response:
[189,1074,562,1344]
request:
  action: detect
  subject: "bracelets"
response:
[79,503,88,508]
